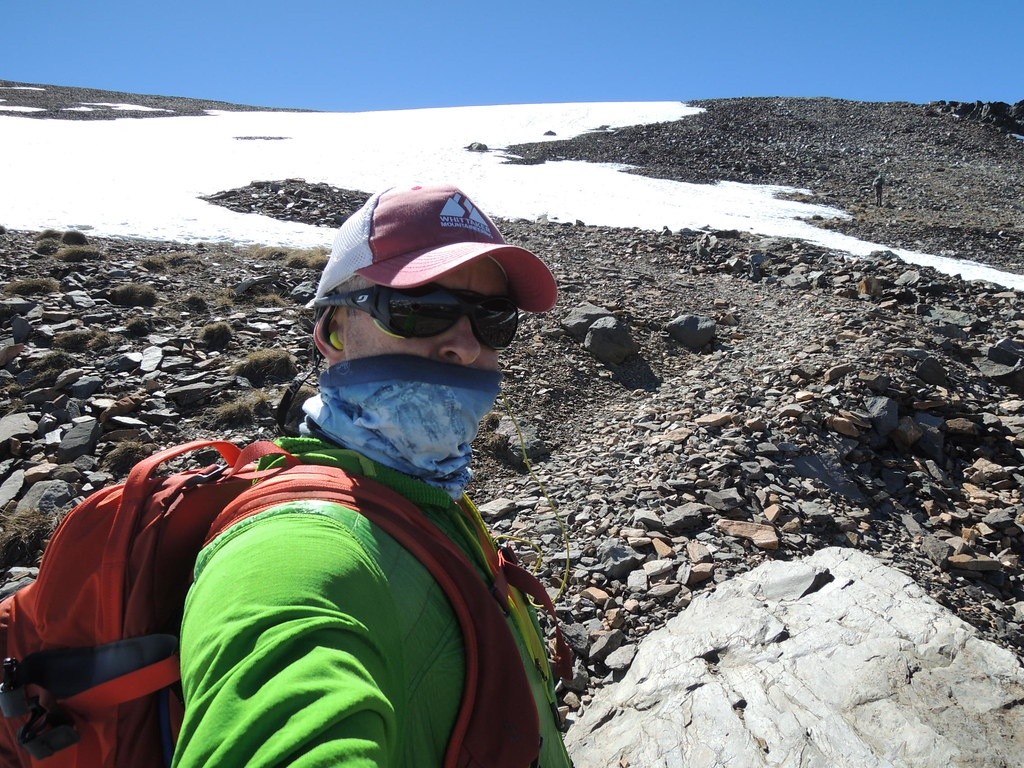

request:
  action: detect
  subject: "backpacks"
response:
[0,439,569,768]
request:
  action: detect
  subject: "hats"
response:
[316,186,557,313]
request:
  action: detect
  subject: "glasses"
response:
[314,280,518,351]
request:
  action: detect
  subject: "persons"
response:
[170,187,574,768]
[405,302,424,333]
